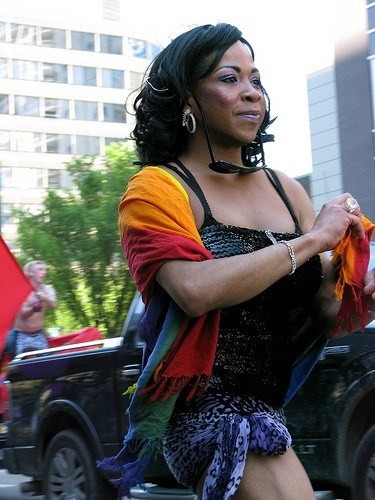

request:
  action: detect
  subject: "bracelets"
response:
[278,240,297,276]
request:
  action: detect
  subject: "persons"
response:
[96,23,374,500]
[8,259,58,362]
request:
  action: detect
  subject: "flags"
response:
[0,235,35,354]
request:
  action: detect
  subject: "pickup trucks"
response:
[2,240,375,495]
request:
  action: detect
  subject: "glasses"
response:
[188,86,267,176]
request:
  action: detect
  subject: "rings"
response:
[346,197,359,214]
